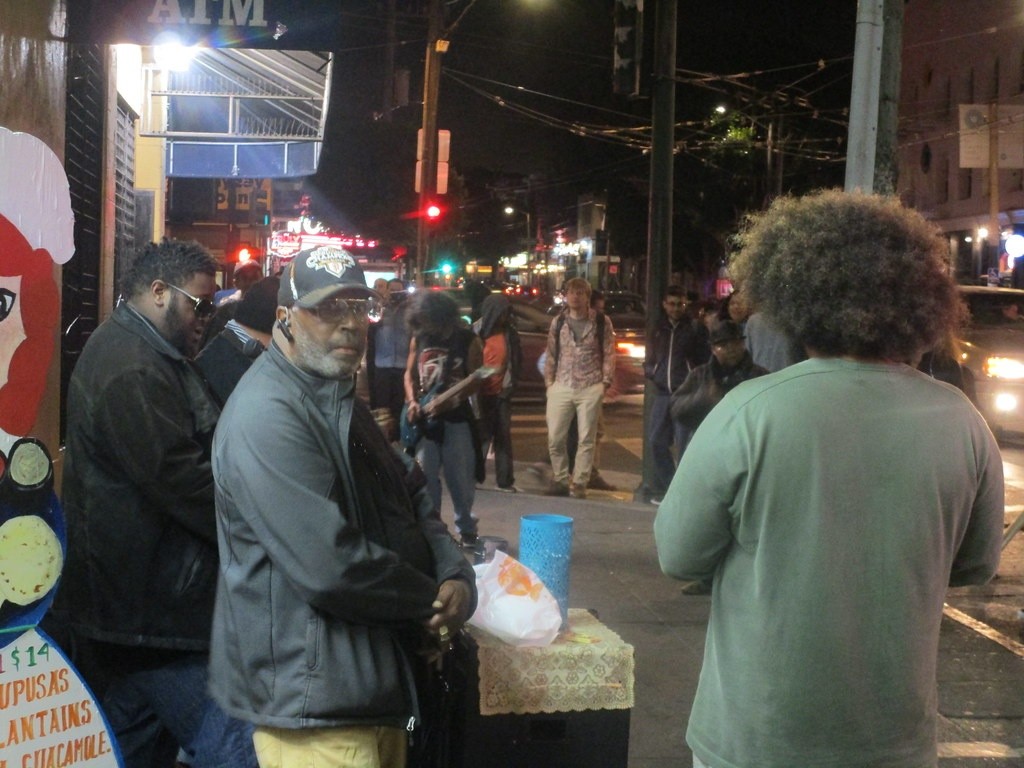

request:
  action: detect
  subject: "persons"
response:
[655,187,1004,768]
[55,235,261,768]
[402,291,485,550]
[192,259,974,596]
[207,246,479,768]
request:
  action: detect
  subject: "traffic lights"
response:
[236,246,255,262]
[426,199,439,221]
[249,188,269,226]
[440,262,453,274]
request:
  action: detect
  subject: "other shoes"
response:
[475,480,486,489]
[495,483,525,492]
[650,494,664,505]
[459,533,478,548]
[551,488,589,496]
[589,476,617,490]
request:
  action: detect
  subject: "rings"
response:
[438,624,455,652]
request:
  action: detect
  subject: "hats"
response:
[235,275,280,335]
[408,292,459,329]
[710,320,746,344]
[233,258,260,276]
[277,246,387,311]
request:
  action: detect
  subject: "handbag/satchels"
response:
[402,624,482,766]
[467,549,563,647]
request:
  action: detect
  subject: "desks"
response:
[457,606,635,767]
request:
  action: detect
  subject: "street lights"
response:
[504,207,530,290]
[715,106,772,203]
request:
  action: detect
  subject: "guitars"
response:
[399,364,497,449]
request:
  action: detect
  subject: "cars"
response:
[448,288,553,395]
[549,292,646,385]
[921,286,1024,436]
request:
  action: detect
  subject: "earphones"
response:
[276,317,293,340]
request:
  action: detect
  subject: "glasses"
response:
[666,301,686,307]
[314,300,386,326]
[165,281,218,321]
